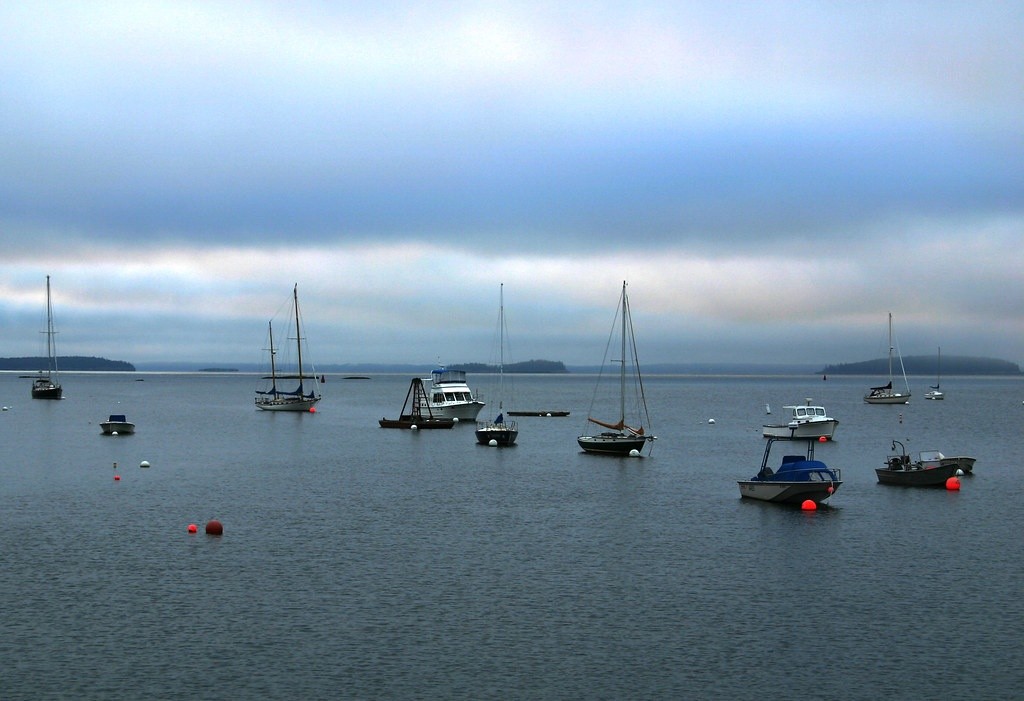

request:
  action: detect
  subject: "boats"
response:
[915,449,976,475]
[507,411,570,416]
[379,377,457,428]
[99,414,136,434]
[761,397,839,441]
[415,368,486,421]
[873,438,958,487]
[736,435,844,504]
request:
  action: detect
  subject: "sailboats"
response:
[923,346,945,399]
[474,282,518,446]
[31,275,63,399]
[863,311,911,404]
[576,280,658,456]
[254,282,322,411]
